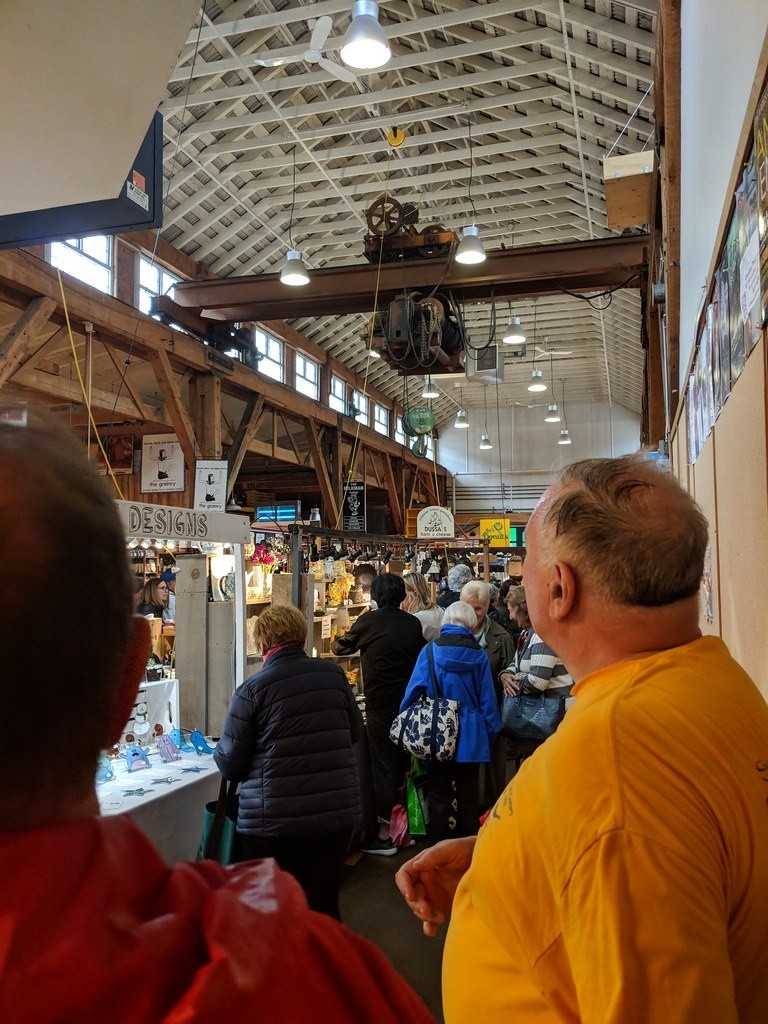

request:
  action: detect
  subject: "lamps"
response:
[479,388,492,449]
[557,378,571,444]
[501,300,526,345]
[422,379,439,398]
[309,504,320,521]
[454,387,470,429]
[310,544,415,565]
[454,115,487,265]
[339,0,392,69]
[527,297,548,392]
[278,144,311,286]
[544,353,562,423]
[226,500,241,510]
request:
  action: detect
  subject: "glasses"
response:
[156,586,169,590]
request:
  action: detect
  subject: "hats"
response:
[447,564,473,592]
[160,571,176,583]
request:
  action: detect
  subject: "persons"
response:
[497,585,577,761]
[137,578,170,622]
[212,601,372,922]
[393,442,768,1024]
[458,581,515,828]
[0,392,442,1024]
[345,564,518,643]
[400,601,500,849]
[128,576,144,613]
[159,570,176,626]
[331,574,428,857]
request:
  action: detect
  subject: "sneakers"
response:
[355,835,399,857]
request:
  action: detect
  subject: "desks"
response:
[95,737,242,867]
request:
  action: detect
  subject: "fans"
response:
[255,16,357,83]
[533,334,573,361]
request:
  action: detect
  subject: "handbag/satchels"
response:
[500,675,566,741]
[194,775,237,865]
[388,640,462,763]
[407,755,430,836]
